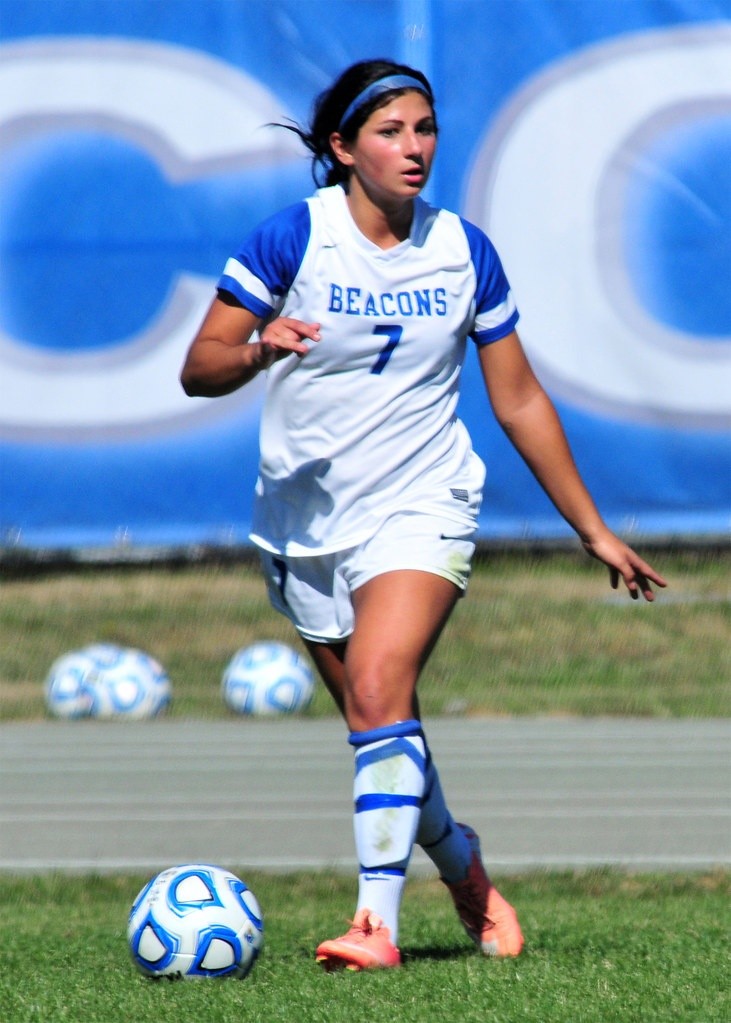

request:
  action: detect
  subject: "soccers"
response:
[42,642,172,722]
[220,642,318,716]
[126,864,264,979]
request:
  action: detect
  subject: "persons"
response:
[178,56,667,969]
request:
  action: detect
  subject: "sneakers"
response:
[312,823,524,974]
